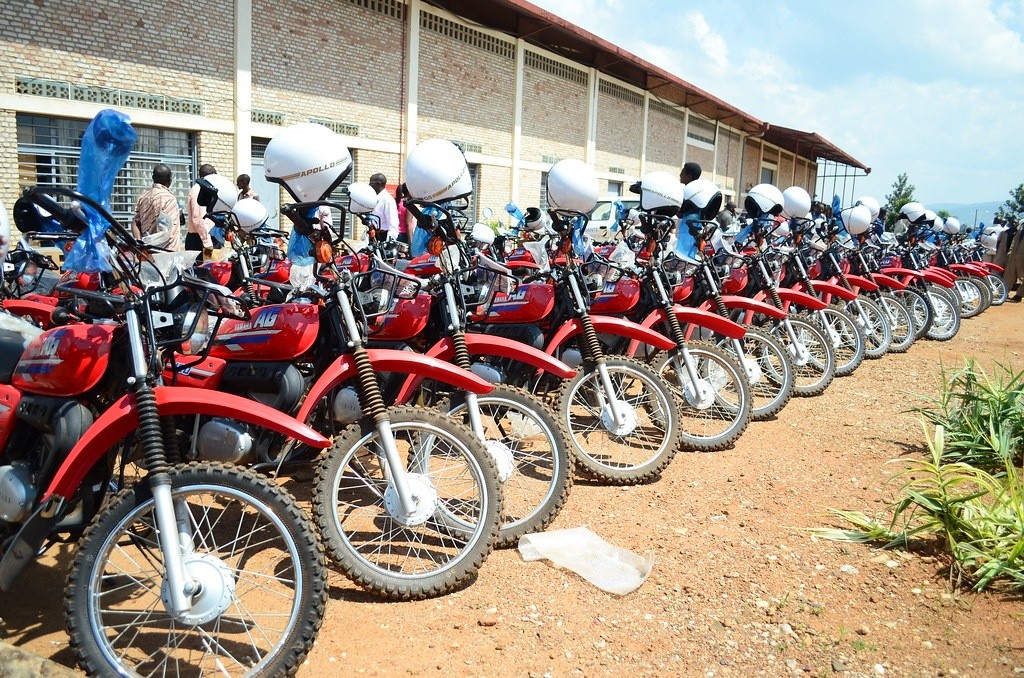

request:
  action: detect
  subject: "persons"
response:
[311,173,415,248]
[679,162,737,233]
[806,201,1024,302]
[133,163,260,251]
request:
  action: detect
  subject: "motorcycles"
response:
[110,202,1009,601]
[1,186,327,677]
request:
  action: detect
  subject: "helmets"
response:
[13,196,45,233]
[898,202,942,232]
[405,137,472,205]
[775,221,789,235]
[231,198,269,232]
[781,186,811,221]
[986,235,997,246]
[815,218,828,232]
[547,158,599,217]
[629,170,684,215]
[265,122,354,213]
[471,223,495,248]
[626,208,640,225]
[195,174,238,215]
[943,217,960,234]
[840,196,880,234]
[681,178,723,220]
[744,184,784,217]
[525,207,546,229]
[725,202,736,209]
[342,182,378,213]
